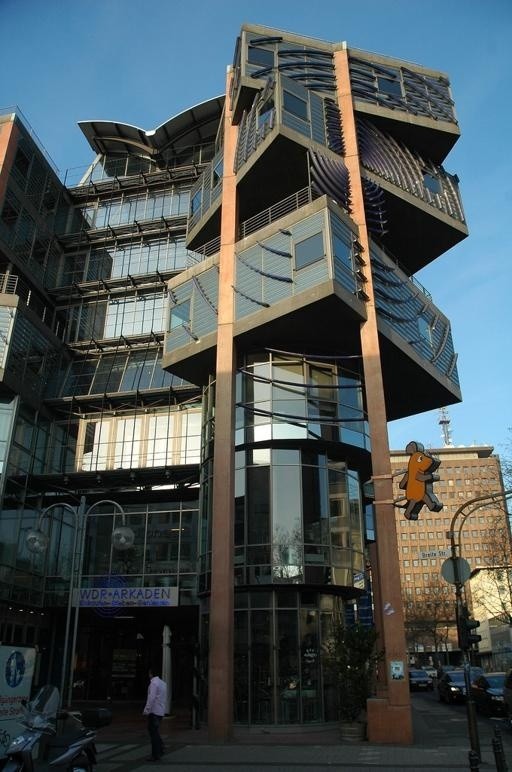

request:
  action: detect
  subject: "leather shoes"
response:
[145,744,165,761]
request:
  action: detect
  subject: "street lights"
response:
[26,496,135,707]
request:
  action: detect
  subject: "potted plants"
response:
[318,621,385,745]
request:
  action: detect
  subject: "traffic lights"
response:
[459,615,481,649]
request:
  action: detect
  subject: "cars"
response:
[409,665,512,732]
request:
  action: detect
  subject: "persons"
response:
[143,666,167,761]
[10,653,17,687]
[392,667,404,680]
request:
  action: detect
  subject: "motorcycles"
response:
[0,699,113,772]
